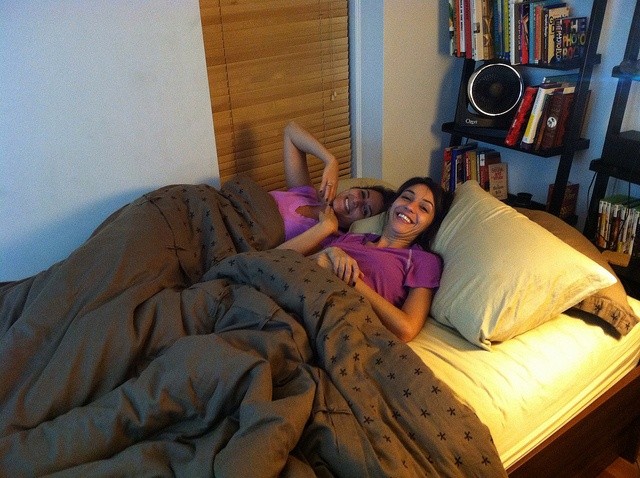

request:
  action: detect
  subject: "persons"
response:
[267,121,396,257]
[305,176,453,344]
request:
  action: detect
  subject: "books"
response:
[592,194,640,261]
[504,72,592,156]
[446,0,588,71]
[546,181,579,220]
[440,141,509,202]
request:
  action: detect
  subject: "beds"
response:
[0,178,640,476]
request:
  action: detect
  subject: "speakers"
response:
[455,56,525,129]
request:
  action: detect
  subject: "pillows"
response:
[317,178,400,234]
[430,179,618,349]
[510,205,639,335]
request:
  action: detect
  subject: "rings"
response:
[326,183,332,185]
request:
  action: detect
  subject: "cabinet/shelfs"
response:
[440,0,609,217]
[584,0,639,298]
